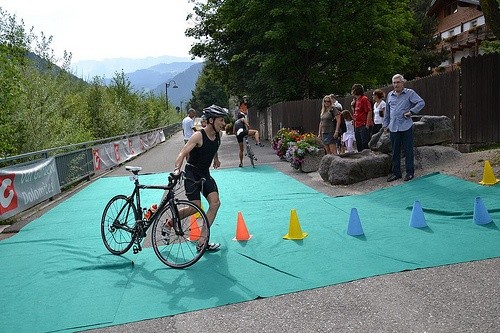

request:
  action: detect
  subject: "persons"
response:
[318,96,341,154]
[182,108,197,160]
[161,104,229,251]
[373,89,386,134]
[383,74,424,182]
[351,84,372,152]
[342,110,356,151]
[330,94,343,154]
[233,112,264,167]
[201,115,206,128]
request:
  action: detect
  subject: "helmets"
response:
[237,111,246,119]
[202,104,229,120]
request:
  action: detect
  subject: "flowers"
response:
[271,126,323,169]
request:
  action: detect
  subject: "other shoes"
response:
[239,163,243,167]
[404,173,414,181]
[255,142,264,147]
[387,174,401,182]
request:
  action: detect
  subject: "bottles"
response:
[141,208,147,220]
[144,205,157,222]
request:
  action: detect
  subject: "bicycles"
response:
[243,133,258,169]
[99,166,211,269]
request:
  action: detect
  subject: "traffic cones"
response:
[188,214,201,241]
[473,195,493,226]
[232,211,253,241]
[408,199,427,229]
[479,160,500,185]
[346,208,365,236]
[282,209,308,240]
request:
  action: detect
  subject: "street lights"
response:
[166,79,180,125]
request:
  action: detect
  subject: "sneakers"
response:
[161,218,172,245]
[196,240,221,251]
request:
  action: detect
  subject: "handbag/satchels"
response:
[379,110,384,117]
[367,127,391,154]
[331,107,347,136]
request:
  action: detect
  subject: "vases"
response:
[300,146,328,173]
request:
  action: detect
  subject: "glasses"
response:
[324,100,330,103]
